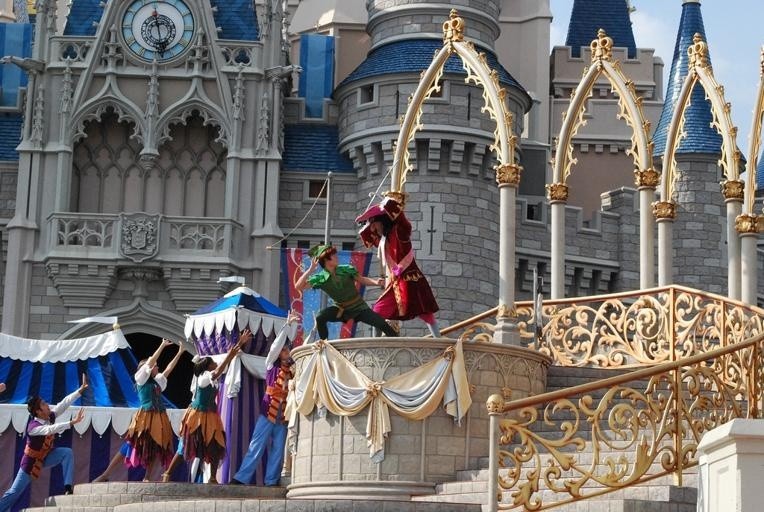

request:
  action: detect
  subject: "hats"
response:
[356,205,387,222]
[308,243,332,259]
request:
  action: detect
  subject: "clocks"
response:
[115,0,198,71]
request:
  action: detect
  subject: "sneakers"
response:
[92,476,108,482]
[163,472,171,482]
[64,487,73,494]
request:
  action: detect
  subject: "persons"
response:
[93,338,185,482]
[355,196,441,337]
[295,244,398,340]
[161,329,252,483]
[0,384,88,511]
[230,309,317,486]
